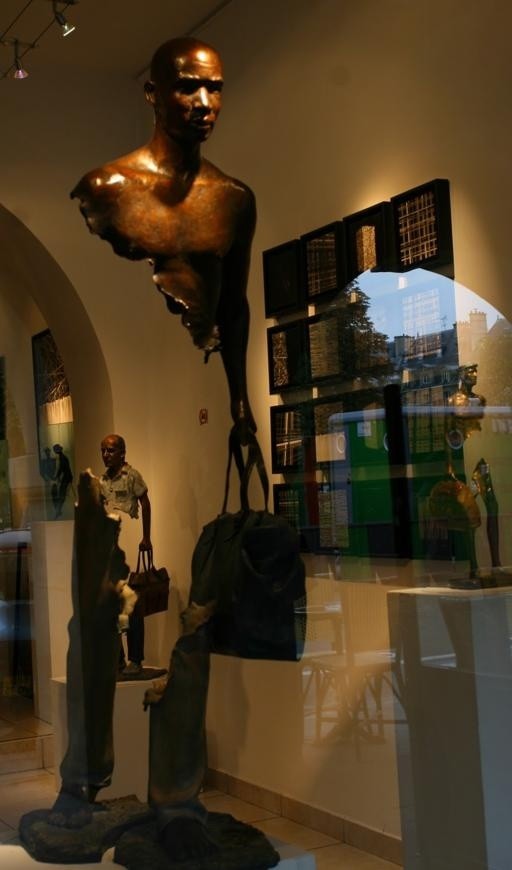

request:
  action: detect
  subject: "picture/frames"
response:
[29,326,79,524]
[264,177,473,562]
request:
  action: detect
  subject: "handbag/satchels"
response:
[125,544,171,619]
[181,421,310,667]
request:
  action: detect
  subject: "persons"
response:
[40,447,56,483]
[446,363,486,434]
[97,434,152,676]
[50,443,73,521]
[45,35,257,861]
